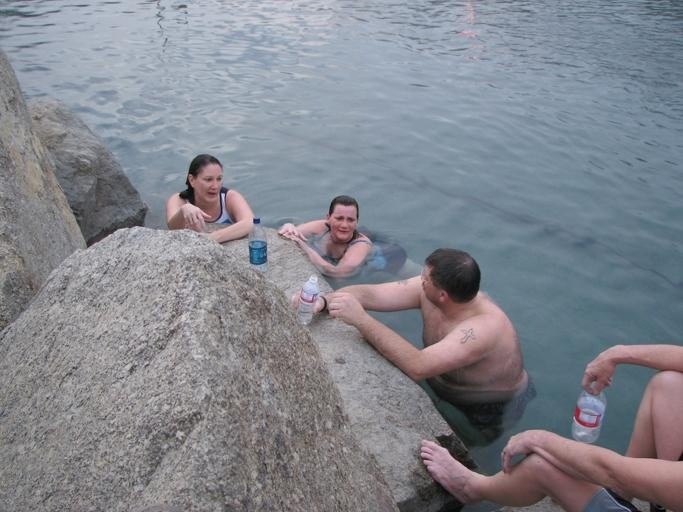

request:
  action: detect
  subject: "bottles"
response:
[295,274,320,326]
[571,381,606,443]
[248,216,268,273]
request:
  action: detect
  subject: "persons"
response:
[418,341,681,512]
[291,247,537,442]
[164,154,254,244]
[275,195,424,282]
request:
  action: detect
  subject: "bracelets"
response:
[318,295,328,312]
